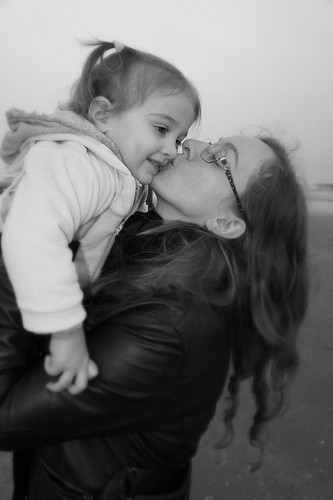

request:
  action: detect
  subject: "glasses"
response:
[200,137,253,233]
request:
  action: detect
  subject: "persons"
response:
[0,135,308,500]
[0,40,201,395]
